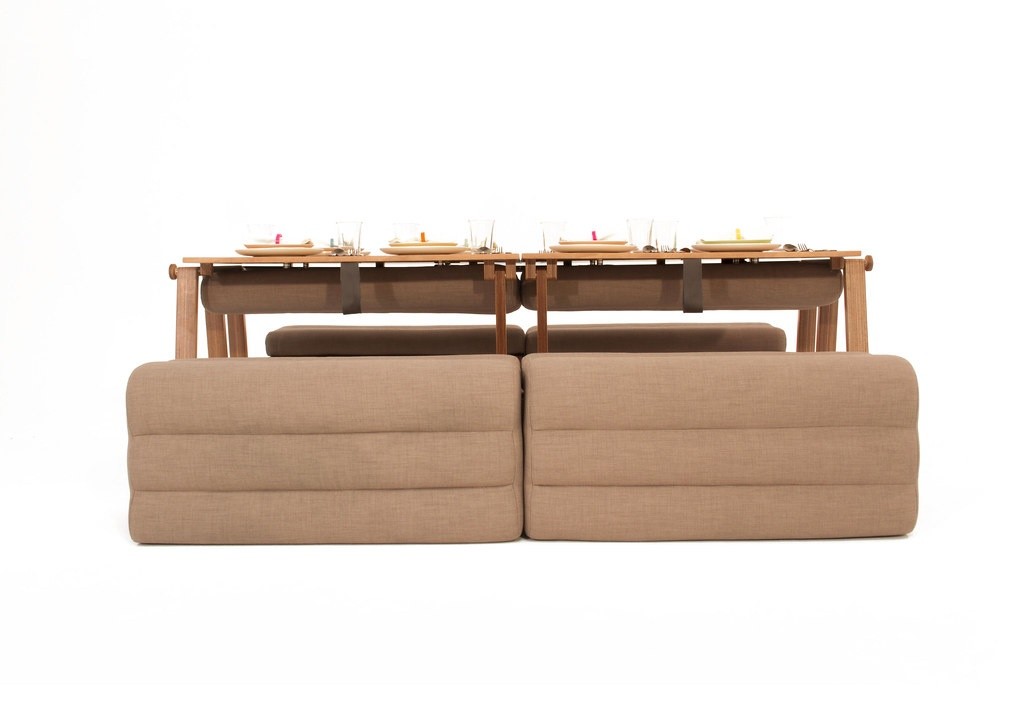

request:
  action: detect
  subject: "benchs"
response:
[126,323,919,544]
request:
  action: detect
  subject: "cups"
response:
[333,220,363,255]
[654,218,677,253]
[543,221,564,253]
[468,217,496,254]
[627,218,654,253]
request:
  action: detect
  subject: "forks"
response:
[495,246,503,254]
[798,244,837,252]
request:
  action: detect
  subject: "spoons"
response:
[783,244,800,251]
[642,245,658,254]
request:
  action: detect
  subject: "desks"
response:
[168,254,521,359]
[521,250,874,353]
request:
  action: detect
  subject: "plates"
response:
[379,246,467,254]
[388,242,458,247]
[558,241,628,245]
[701,239,773,243]
[692,243,782,251]
[244,243,314,247]
[235,248,324,255]
[549,245,637,252]
